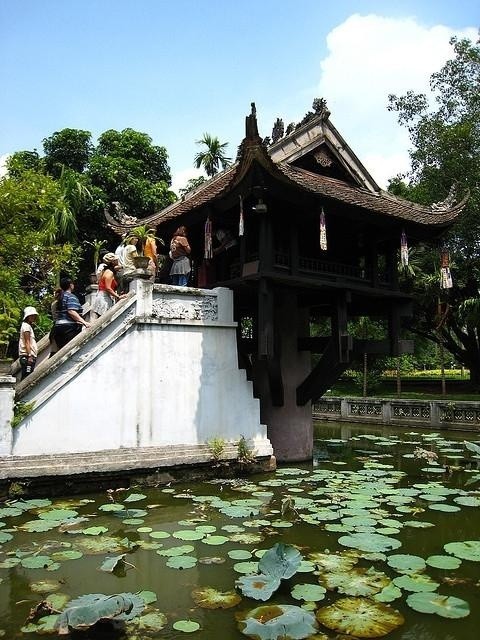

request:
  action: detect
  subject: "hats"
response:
[103,253,119,265]
[23,306,39,320]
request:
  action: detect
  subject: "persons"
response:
[54,277,91,348]
[91,224,160,322]
[18,305,40,382]
[50,288,62,358]
[169,226,193,287]
